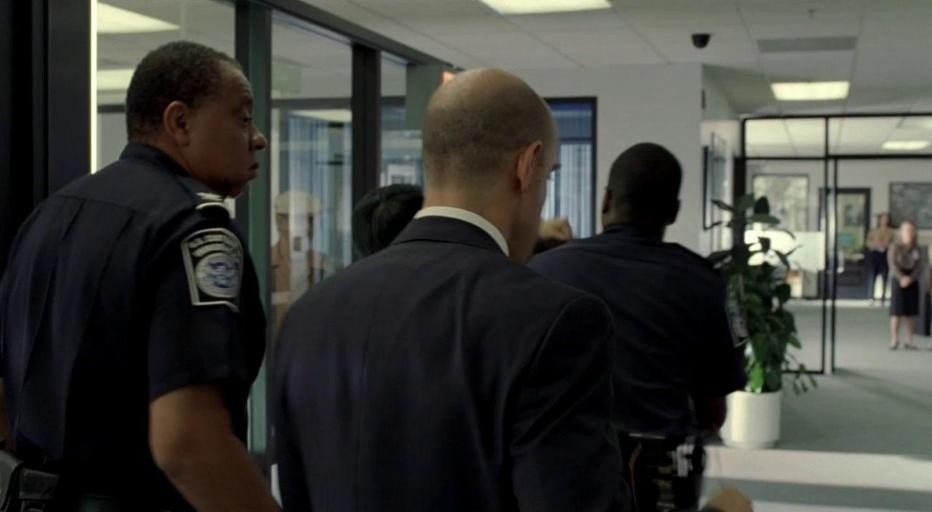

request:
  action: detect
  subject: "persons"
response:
[268,66,755,511]
[349,183,424,259]
[534,213,572,254]
[526,139,748,510]
[884,218,925,353]
[865,211,897,304]
[269,184,337,442]
[2,41,287,512]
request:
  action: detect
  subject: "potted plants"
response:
[705,194,818,450]
